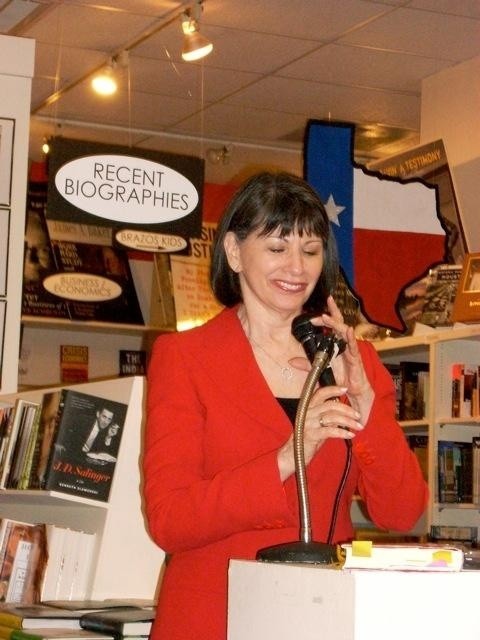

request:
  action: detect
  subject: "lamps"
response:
[208,141,236,166]
[177,4,216,61]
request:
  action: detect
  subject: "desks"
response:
[226,552,474,639]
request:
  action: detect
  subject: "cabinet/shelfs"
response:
[18,313,177,395]
[0,371,153,640]
[348,324,480,542]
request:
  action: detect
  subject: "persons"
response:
[56,403,120,456]
[144,170,430,640]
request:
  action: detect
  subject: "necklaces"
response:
[247,332,302,381]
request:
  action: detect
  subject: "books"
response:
[338,541,465,573]
[451,251,480,325]
[60,345,89,382]
[1,518,156,640]
[450,364,480,419]
[382,359,430,421]
[436,436,480,508]
[0,389,128,503]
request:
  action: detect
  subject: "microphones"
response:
[291,308,354,445]
